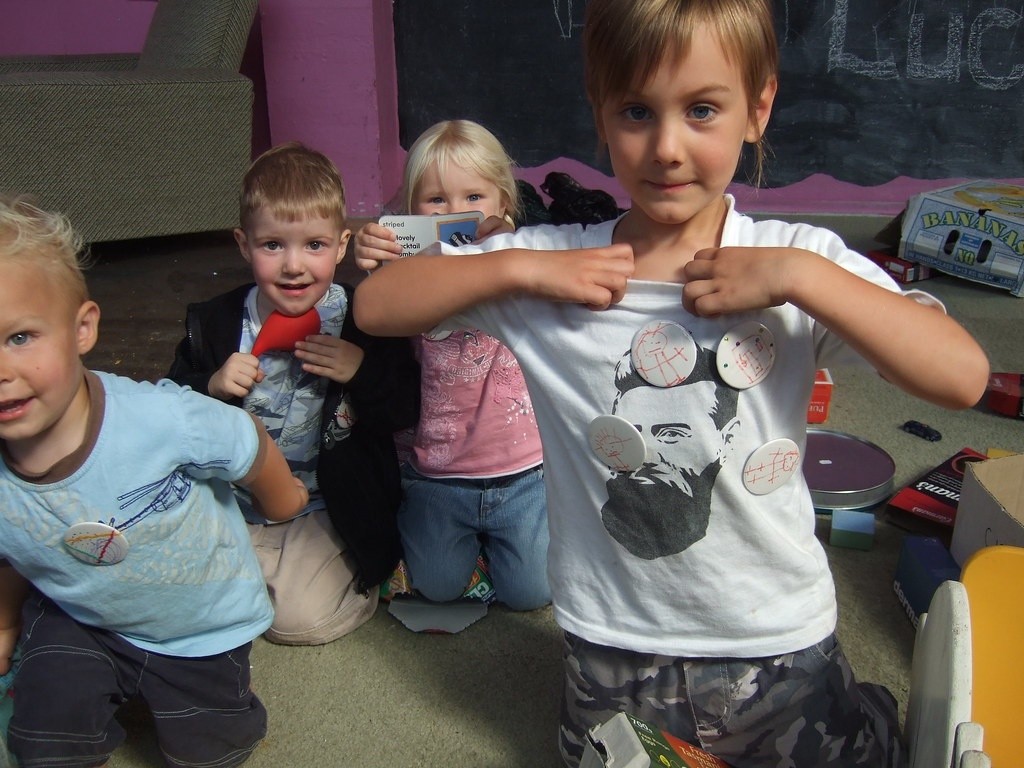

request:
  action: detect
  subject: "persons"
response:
[354,117,551,612]
[350,0,993,764]
[0,197,311,768]
[163,141,422,647]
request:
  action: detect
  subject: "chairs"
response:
[0,0,256,245]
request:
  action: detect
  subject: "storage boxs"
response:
[899,179,1023,297]
[950,454,1023,570]
